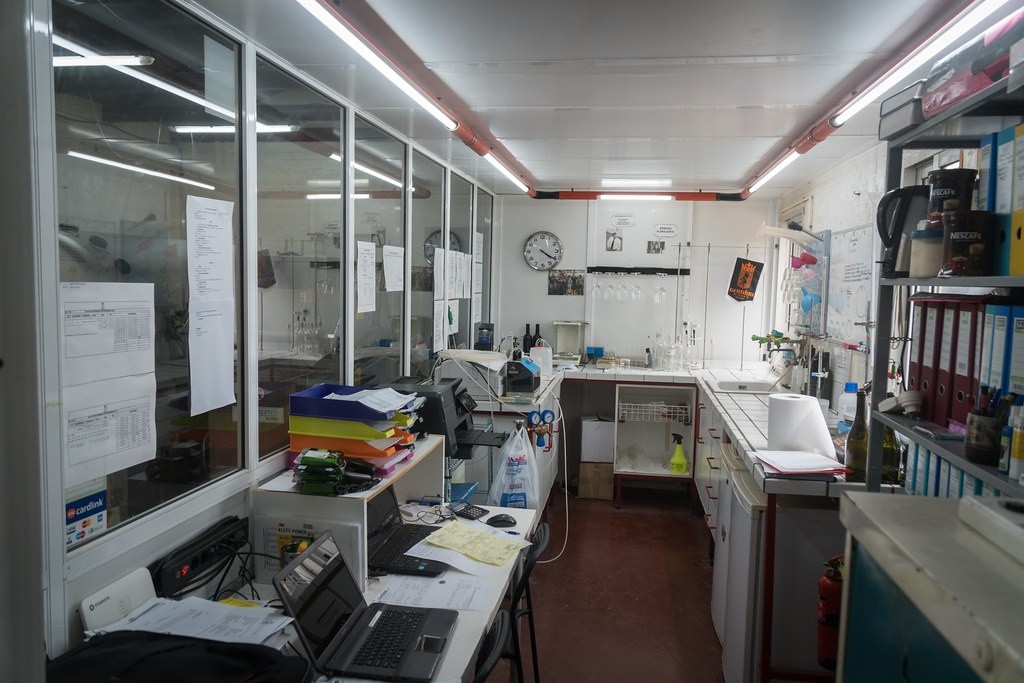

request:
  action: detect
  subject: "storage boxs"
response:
[576,460,615,499]
[578,413,617,463]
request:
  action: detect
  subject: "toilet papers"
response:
[767,392,837,461]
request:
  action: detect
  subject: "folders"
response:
[905,291,1024,435]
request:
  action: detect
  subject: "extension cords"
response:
[152,516,247,599]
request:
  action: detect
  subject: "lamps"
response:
[51,31,434,209]
[290,0,1007,202]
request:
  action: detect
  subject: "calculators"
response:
[446,500,490,520]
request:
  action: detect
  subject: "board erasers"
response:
[842,342,858,350]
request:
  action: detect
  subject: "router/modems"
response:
[79,568,156,630]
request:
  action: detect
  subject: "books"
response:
[877,80,927,144]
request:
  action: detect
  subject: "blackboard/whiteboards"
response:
[825,225,874,356]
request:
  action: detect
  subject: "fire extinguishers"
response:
[813,554,847,672]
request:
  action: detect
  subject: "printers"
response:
[370,376,510,460]
[434,349,540,403]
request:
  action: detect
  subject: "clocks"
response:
[522,230,564,273]
[422,229,461,268]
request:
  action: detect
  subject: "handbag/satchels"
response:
[484,426,539,517]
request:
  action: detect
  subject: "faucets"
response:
[769,348,798,367]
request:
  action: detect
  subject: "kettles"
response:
[875,185,932,278]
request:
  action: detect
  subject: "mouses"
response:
[487,514,517,527]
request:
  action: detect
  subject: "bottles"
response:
[837,382,858,437]
[879,392,901,485]
[999,405,1019,476]
[845,392,869,482]
[792,342,833,410]
[523,324,533,355]
[533,324,542,348]
[1009,405,1024,481]
[649,321,702,372]
[289,311,326,356]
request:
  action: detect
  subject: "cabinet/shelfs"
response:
[612,383,699,514]
[530,380,561,535]
[693,386,714,518]
[706,412,725,541]
[859,65,1024,505]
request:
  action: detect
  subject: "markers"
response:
[858,344,867,349]
[857,347,866,351]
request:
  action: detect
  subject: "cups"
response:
[965,412,1007,467]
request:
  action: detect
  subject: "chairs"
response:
[466,609,512,682]
[488,520,549,683]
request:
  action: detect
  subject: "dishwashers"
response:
[710,444,769,683]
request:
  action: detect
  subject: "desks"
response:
[220,503,539,683]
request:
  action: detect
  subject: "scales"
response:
[551,320,585,367]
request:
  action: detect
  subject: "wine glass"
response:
[629,273,641,302]
[591,271,602,300]
[604,272,616,301]
[616,272,628,301]
[653,272,668,304]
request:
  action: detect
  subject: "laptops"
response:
[366,483,450,576]
[273,530,458,682]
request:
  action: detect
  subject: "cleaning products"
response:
[670,432,689,475]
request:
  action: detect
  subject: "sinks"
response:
[705,377,782,395]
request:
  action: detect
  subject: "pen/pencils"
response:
[979,384,1017,420]
[503,529,520,536]
